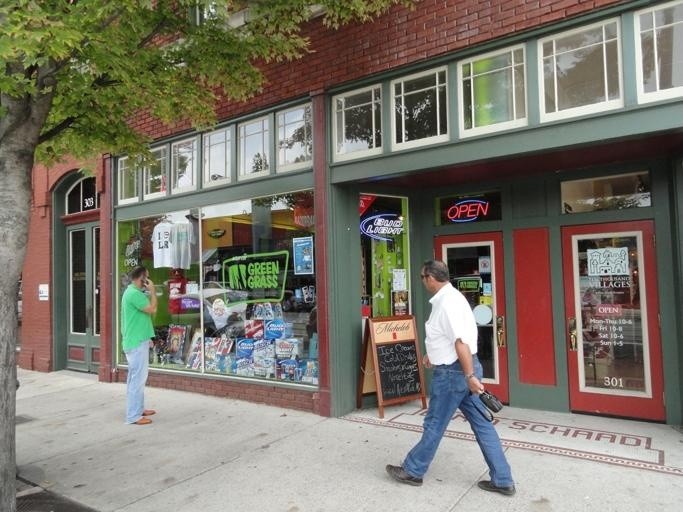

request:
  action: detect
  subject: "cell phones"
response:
[140,274,149,284]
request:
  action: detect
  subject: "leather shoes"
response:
[386,465,423,486]
[478,480,516,495]
[137,418,151,424]
[143,410,156,416]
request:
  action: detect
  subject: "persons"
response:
[385,260,517,496]
[119,265,158,426]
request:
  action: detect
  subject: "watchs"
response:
[464,373,474,383]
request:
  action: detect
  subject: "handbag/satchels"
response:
[479,390,503,413]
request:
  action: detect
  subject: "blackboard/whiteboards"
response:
[373,339,424,406]
